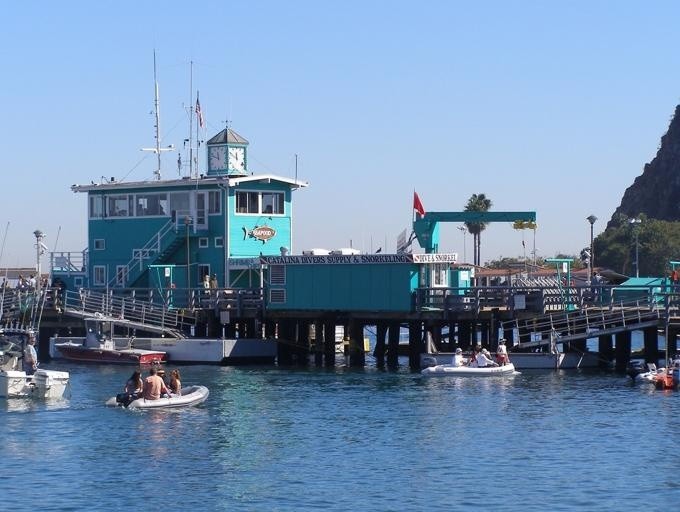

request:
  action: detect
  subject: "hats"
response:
[157,370,165,374]
[498,338,507,343]
[456,348,462,353]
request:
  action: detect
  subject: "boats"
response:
[421,361,516,381]
[0,368,70,402]
[636,366,673,382]
[105,382,209,412]
[50,316,170,368]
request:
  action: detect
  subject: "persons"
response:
[113,204,165,215]
[0,273,73,337]
[211,273,218,307]
[673,354,680,367]
[561,275,575,287]
[671,267,680,292]
[116,364,182,409]
[592,271,599,294]
[203,274,210,307]
[452,338,510,368]
[24,336,39,382]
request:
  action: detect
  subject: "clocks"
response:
[206,144,227,173]
[230,144,247,172]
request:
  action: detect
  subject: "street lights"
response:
[628,215,642,278]
[586,214,599,298]
[33,231,45,310]
[456,224,467,263]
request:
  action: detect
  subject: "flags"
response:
[412,191,425,216]
[195,93,204,129]
